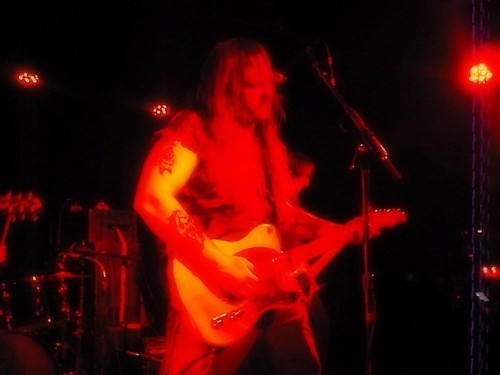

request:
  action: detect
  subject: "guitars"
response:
[168,206,407,350]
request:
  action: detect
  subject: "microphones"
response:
[305,46,337,88]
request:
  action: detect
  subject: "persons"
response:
[131,40,380,375]
